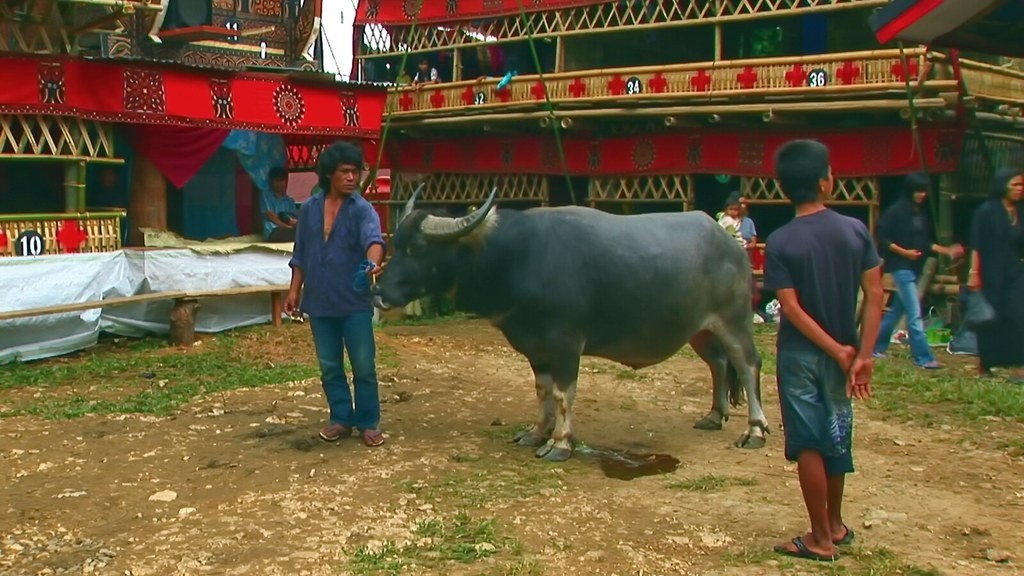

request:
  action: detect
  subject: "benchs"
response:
[0,285,304,344]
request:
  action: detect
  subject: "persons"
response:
[89,163,126,246]
[284,143,388,447]
[394,54,443,90]
[476,75,494,83]
[259,165,298,243]
[966,165,1024,382]
[715,191,765,324]
[873,171,964,368]
[764,139,884,561]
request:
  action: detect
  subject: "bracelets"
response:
[969,268,980,276]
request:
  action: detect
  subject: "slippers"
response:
[774,536,841,561]
[832,522,854,545]
[319,423,353,441]
[363,429,384,447]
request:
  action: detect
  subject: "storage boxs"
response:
[928,328,951,344]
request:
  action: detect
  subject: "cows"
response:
[368,179,772,465]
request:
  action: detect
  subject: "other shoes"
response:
[914,358,947,369]
[872,352,886,358]
[753,313,764,323]
